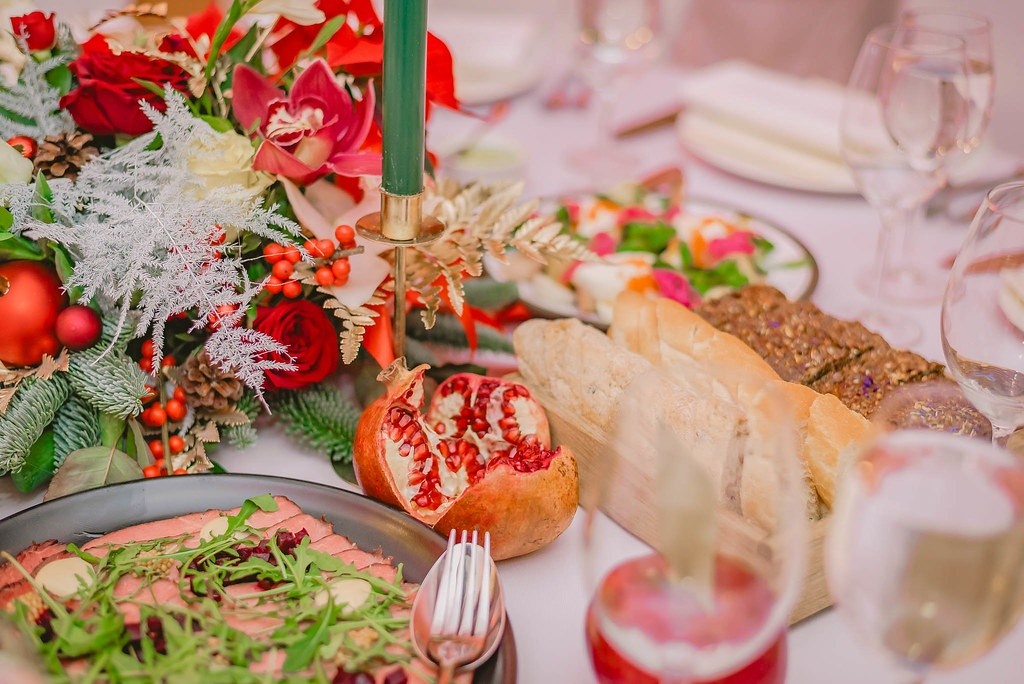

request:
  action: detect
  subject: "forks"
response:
[428,527,491,684]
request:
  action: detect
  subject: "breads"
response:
[505,283,1024,550]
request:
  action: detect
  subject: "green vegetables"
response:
[0,492,446,684]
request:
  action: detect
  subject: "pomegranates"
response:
[350,357,579,562]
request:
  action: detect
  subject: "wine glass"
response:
[834,6,995,315]
[939,180,1024,454]
[564,1,686,176]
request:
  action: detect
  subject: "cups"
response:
[825,382,1024,683]
[583,366,809,682]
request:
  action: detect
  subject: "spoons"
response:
[409,541,506,684]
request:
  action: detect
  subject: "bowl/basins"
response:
[0,470,519,684]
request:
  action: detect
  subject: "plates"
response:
[672,78,894,192]
[480,190,817,328]
[448,46,539,108]
